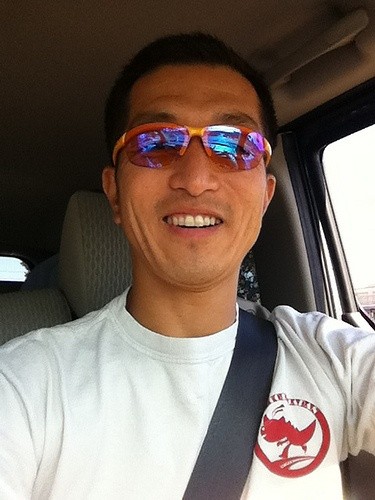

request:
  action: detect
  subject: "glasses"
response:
[111,120,273,172]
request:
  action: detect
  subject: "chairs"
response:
[0,191,133,347]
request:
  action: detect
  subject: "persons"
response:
[0,30,375,500]
